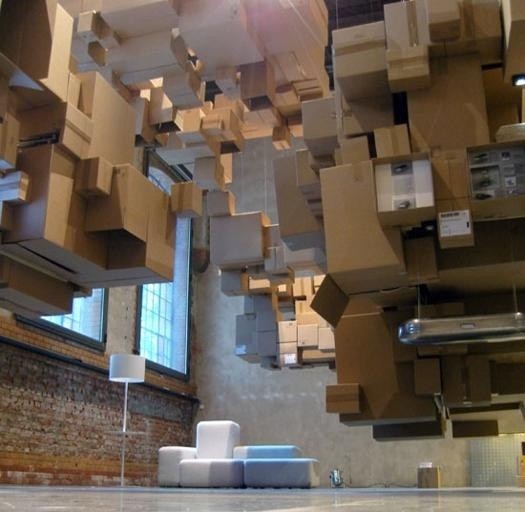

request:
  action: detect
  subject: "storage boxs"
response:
[2,2,523,440]
